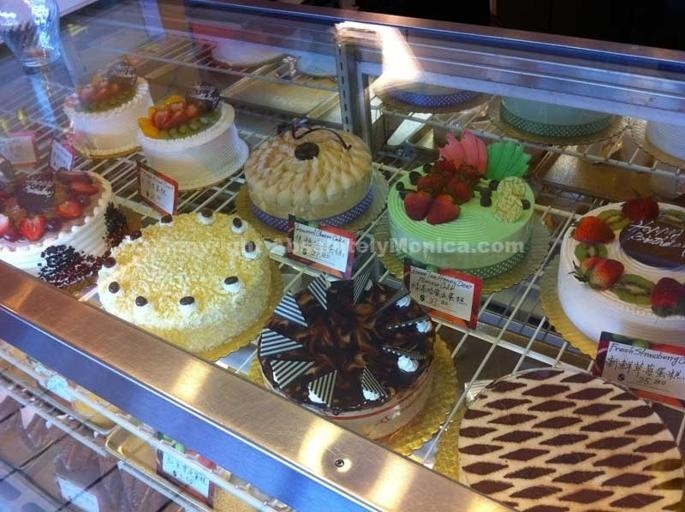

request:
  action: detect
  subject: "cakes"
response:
[243,118,373,220]
[97,209,272,355]
[557,197,685,348]
[257,270,437,440]
[457,367,685,512]
[136,80,241,185]
[388,130,536,271]
[62,61,154,150]
[0,166,128,290]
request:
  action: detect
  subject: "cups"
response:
[1,0,63,74]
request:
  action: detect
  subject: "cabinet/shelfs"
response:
[1,0,685,511]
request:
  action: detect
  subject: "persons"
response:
[335,21,423,100]
